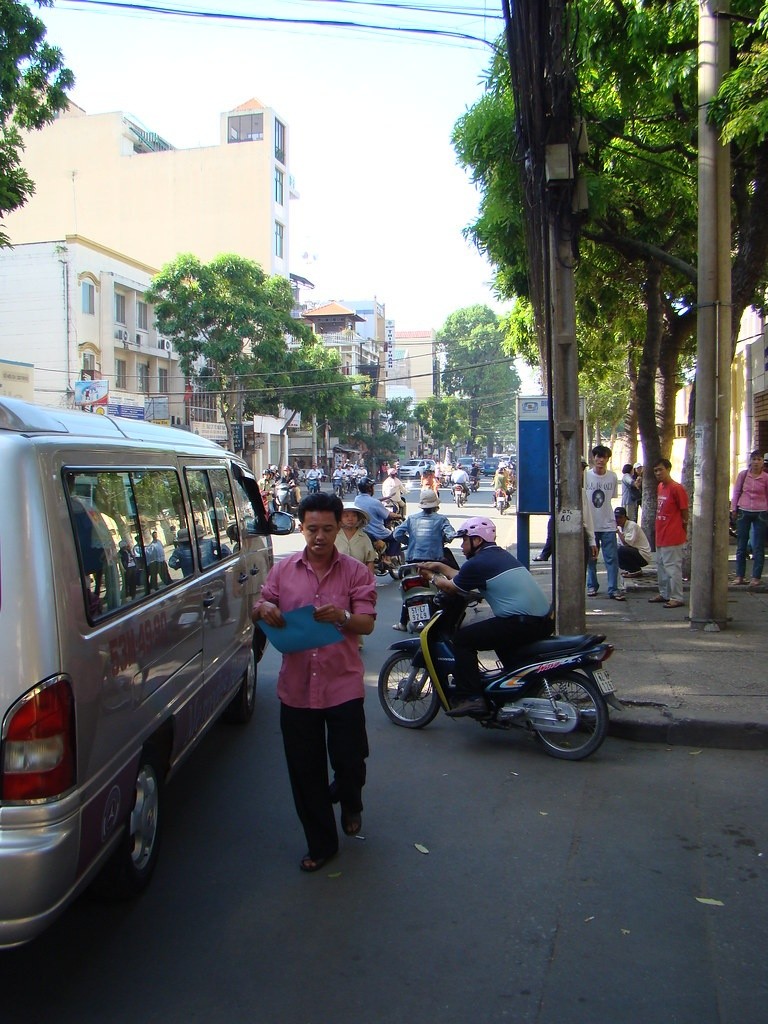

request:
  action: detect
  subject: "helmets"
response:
[387,468,396,476]
[357,477,375,493]
[472,463,476,467]
[498,468,504,473]
[453,516,496,543]
[457,463,462,469]
[262,462,364,475]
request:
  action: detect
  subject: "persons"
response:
[251,491,379,874]
[414,516,555,718]
[621,462,644,523]
[730,450,768,586]
[490,460,516,507]
[226,524,272,640]
[331,459,368,493]
[118,518,237,627]
[381,462,389,480]
[584,446,626,602]
[469,462,481,485]
[420,464,440,498]
[581,455,598,586]
[648,459,688,608]
[451,462,471,503]
[614,506,651,577]
[381,469,410,520]
[256,457,324,516]
[532,514,552,561]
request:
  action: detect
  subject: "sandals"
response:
[340,797,362,835]
[301,852,334,872]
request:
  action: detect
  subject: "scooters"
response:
[302,470,320,494]
[344,474,362,496]
[329,472,345,499]
[260,483,293,516]
[397,535,460,633]
[451,486,469,507]
[437,477,449,487]
[382,490,406,521]
[373,517,406,580]
[468,476,480,492]
[377,588,623,761]
[491,477,517,515]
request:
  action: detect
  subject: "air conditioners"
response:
[160,338,172,352]
[118,329,129,341]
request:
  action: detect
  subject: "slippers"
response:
[613,593,626,600]
[532,557,544,561]
[588,589,596,595]
[393,624,407,631]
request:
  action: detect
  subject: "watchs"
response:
[338,610,350,626]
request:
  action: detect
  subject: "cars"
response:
[399,459,436,479]
[475,454,517,477]
[455,458,478,475]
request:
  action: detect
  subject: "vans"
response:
[0,397,295,951]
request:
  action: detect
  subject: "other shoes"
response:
[649,595,665,601]
[663,600,682,608]
[444,697,488,717]
[621,571,642,577]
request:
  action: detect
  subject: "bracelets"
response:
[429,573,437,585]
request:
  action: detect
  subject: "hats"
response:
[340,504,369,529]
[177,530,189,542]
[614,507,626,516]
[633,463,642,469]
[419,490,440,509]
[196,525,205,540]
